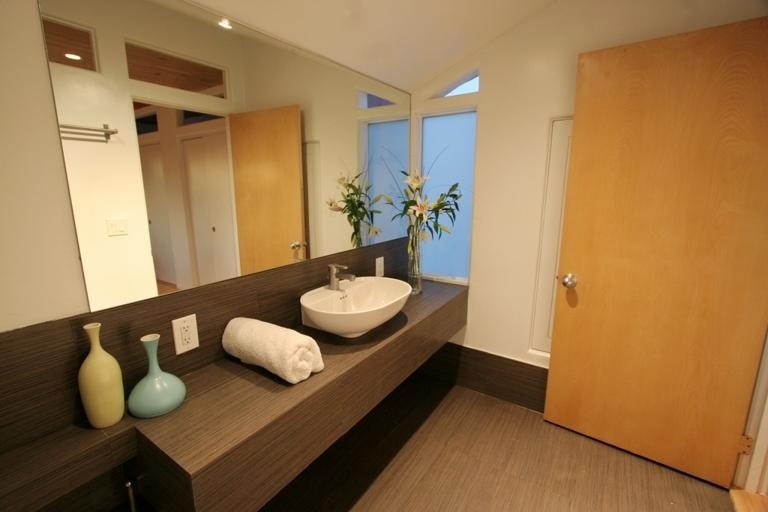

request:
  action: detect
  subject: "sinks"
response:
[299,276,413,338]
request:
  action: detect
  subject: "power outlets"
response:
[171,313,199,355]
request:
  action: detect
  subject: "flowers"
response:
[324,167,393,245]
[370,148,462,239]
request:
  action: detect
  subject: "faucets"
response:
[328,263,355,290]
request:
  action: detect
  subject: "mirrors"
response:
[1,0,412,334]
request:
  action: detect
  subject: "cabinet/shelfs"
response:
[136,279,469,512]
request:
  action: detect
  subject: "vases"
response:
[77,322,125,429]
[406,238,423,295]
[127,334,187,420]
